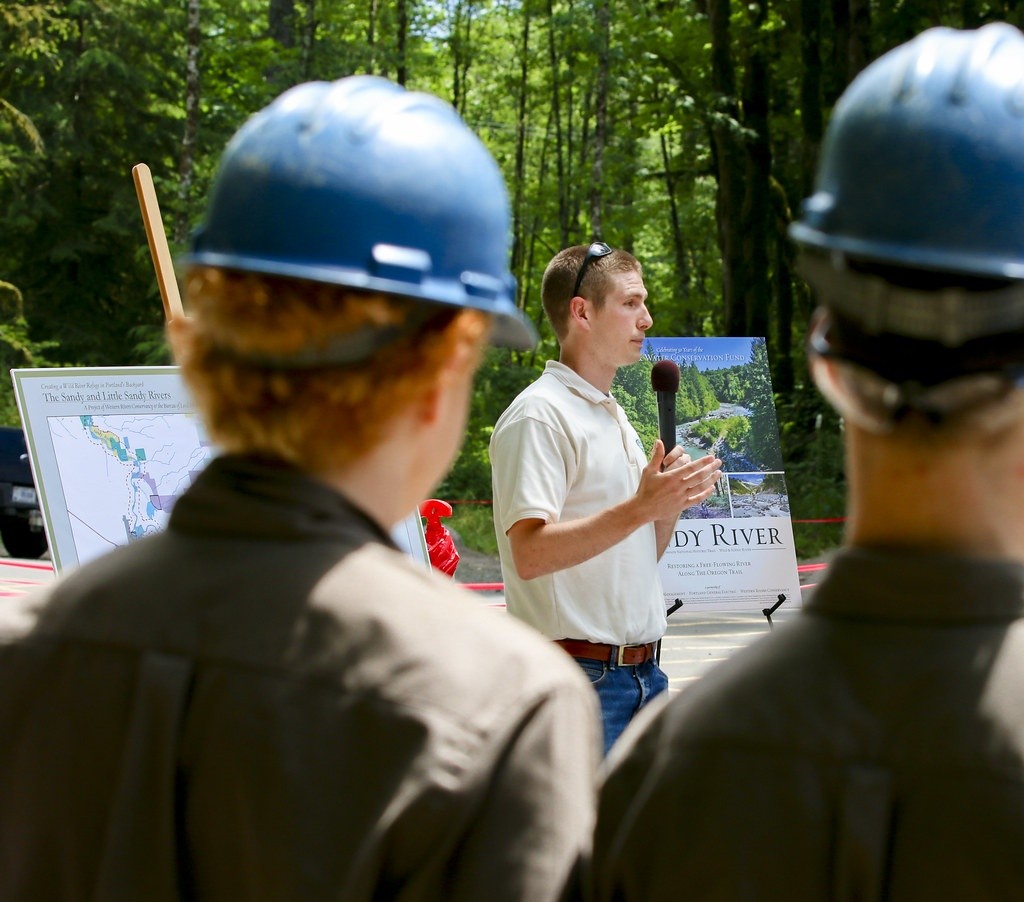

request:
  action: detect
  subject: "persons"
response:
[560,23,1024,902]
[488,244,721,761]
[0,79,600,902]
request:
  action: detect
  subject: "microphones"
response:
[651,360,680,457]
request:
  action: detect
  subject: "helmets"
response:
[787,20,1024,276]
[185,76,541,352]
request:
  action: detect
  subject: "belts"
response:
[555,639,663,668]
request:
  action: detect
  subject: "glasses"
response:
[572,241,612,301]
